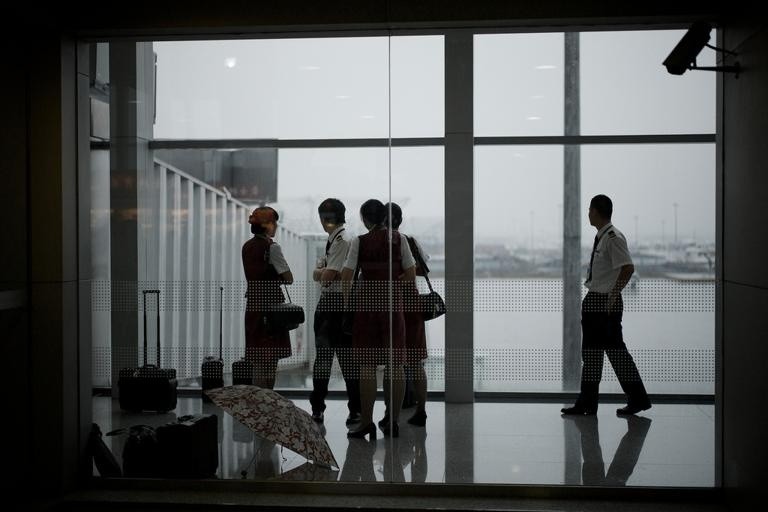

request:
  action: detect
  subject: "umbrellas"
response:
[203,384,340,478]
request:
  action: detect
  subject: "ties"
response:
[324,229,345,268]
[588,226,611,280]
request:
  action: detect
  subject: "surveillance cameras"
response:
[662,20,743,80]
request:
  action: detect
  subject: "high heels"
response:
[408,409,426,426]
[349,423,377,441]
[402,395,410,407]
[379,412,399,438]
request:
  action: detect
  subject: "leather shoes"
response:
[346,413,360,425]
[616,401,652,418]
[312,411,324,423]
[561,404,598,416]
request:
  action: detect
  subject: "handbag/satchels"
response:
[407,236,447,322]
[327,230,366,349]
[264,241,305,334]
[106,414,218,479]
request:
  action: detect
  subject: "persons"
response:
[384,202,429,427]
[311,199,361,426]
[242,207,293,390]
[560,196,652,416]
[341,199,416,441]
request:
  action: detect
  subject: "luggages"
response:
[201,289,223,403]
[119,291,178,412]
[232,358,252,386]
